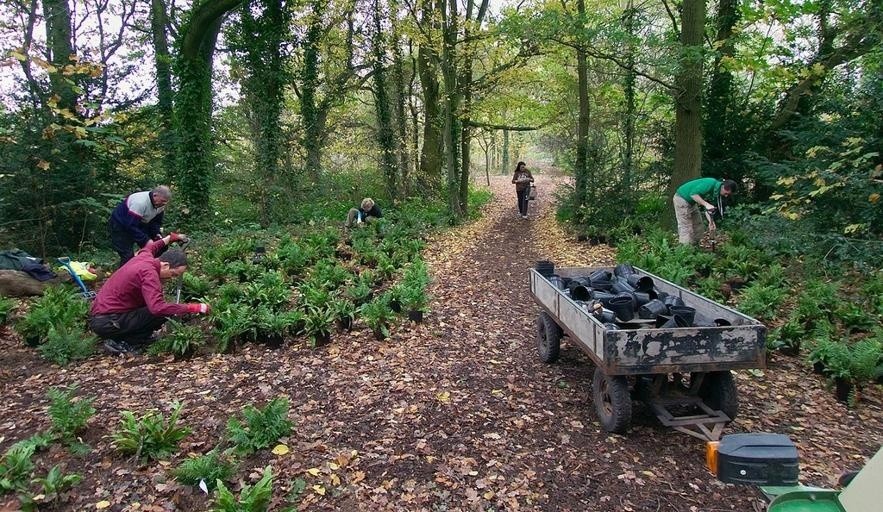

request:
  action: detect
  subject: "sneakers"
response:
[103,339,149,357]
[517,211,528,219]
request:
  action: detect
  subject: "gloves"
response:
[168,230,189,247]
[186,301,210,317]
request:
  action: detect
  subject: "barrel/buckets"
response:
[533,257,733,328]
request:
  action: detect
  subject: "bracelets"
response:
[166,232,178,244]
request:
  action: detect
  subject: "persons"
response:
[84,232,210,355]
[109,183,173,269]
[672,176,739,246]
[342,196,383,229]
[511,161,535,218]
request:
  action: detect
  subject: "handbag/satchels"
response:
[524,179,537,201]
